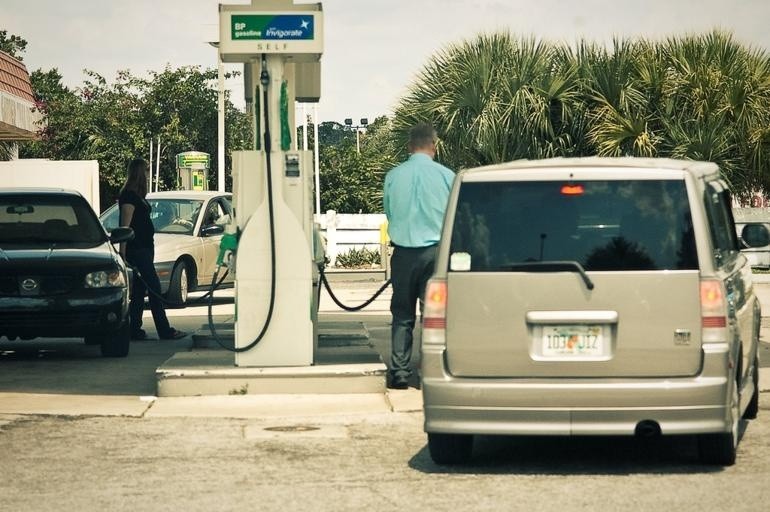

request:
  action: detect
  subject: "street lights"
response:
[345,115,370,155]
[203,20,230,192]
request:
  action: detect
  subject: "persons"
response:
[380,122,459,390]
[116,157,189,341]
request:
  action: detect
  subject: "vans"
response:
[417,152,764,477]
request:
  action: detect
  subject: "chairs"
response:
[44,218,70,240]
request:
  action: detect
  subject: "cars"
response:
[89,190,239,312]
[1,187,137,363]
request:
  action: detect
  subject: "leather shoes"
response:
[386,380,409,390]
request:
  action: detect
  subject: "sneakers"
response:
[168,329,188,340]
